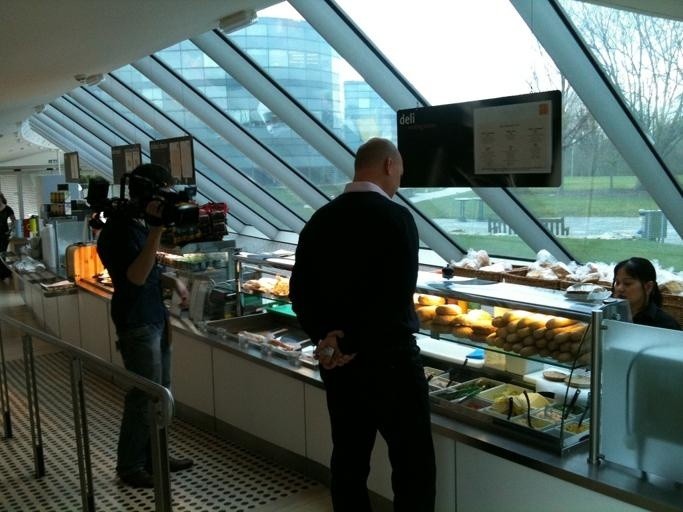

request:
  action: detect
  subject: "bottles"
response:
[15,218,36,239]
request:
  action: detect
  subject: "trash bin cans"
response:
[638,209,667,243]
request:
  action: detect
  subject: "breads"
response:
[414,294,591,364]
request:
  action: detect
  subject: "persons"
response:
[610,255,683,334]
[285,136,438,512]
[93,162,196,489]
[0,192,15,281]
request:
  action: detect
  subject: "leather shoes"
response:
[169,452,192,473]
[124,470,155,490]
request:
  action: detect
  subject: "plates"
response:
[543,370,591,389]
[101,278,113,287]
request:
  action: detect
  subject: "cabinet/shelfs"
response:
[0,220,683,512]
